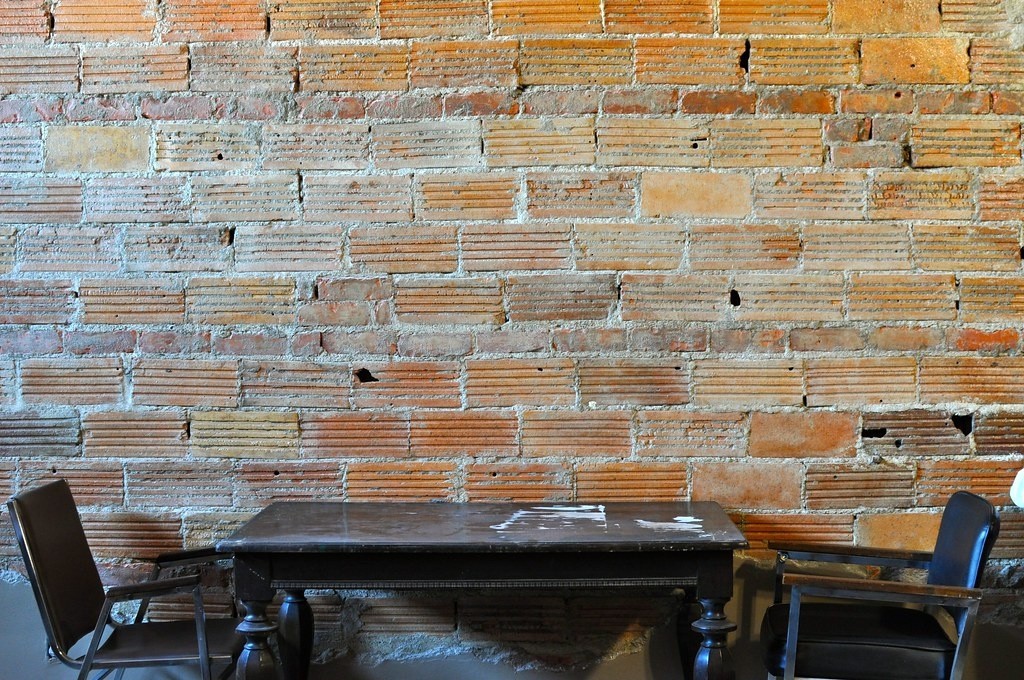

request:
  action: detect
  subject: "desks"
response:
[218,500,748,680]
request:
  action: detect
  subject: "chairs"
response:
[8,478,246,680]
[758,489,1001,680]
